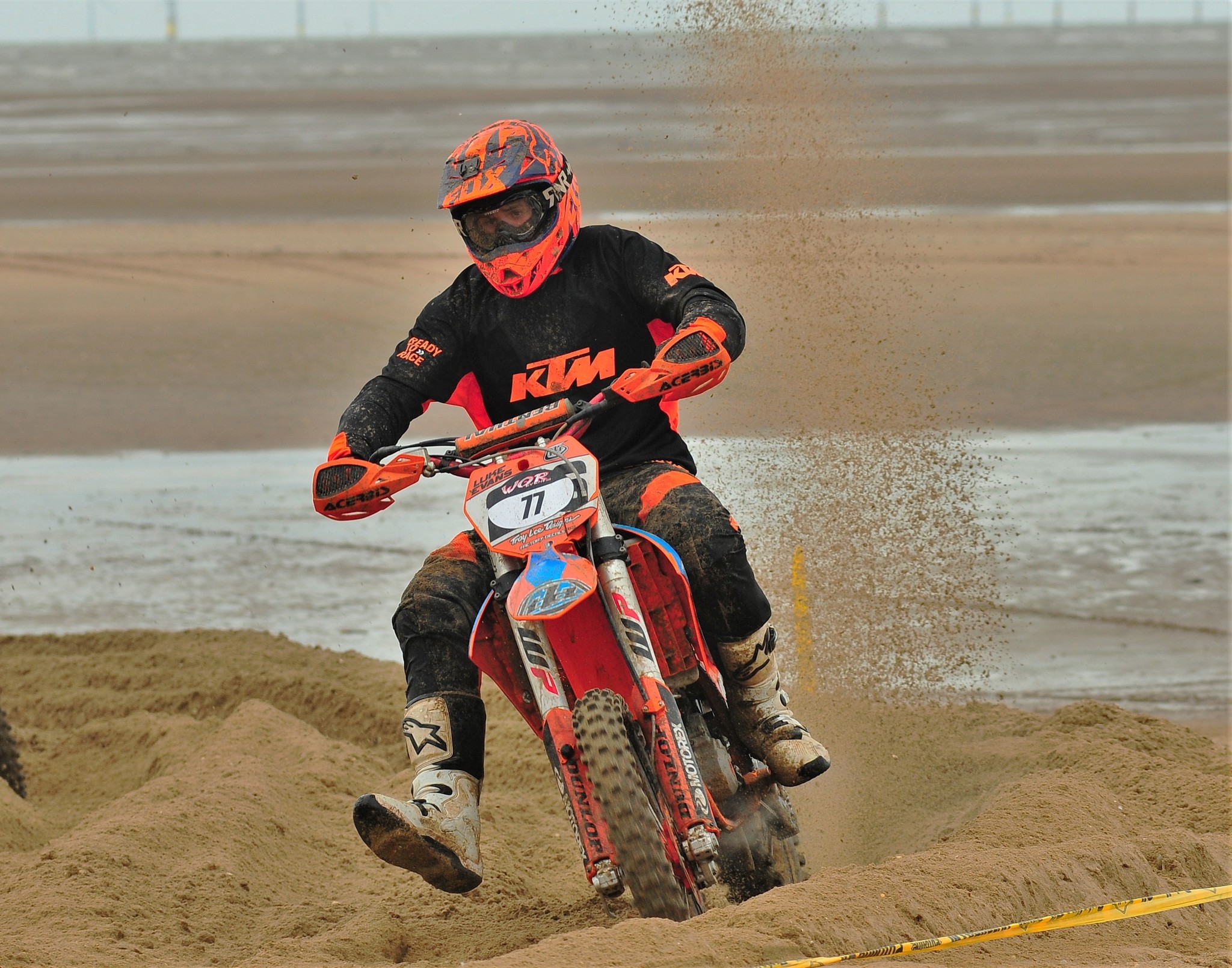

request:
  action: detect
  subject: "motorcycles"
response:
[312,326,811,922]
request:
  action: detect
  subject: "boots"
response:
[708,623,832,790]
[353,690,487,896]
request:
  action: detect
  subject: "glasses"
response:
[459,188,553,250]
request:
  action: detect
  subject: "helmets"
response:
[440,119,581,299]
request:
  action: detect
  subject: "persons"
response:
[311,120,831,893]
